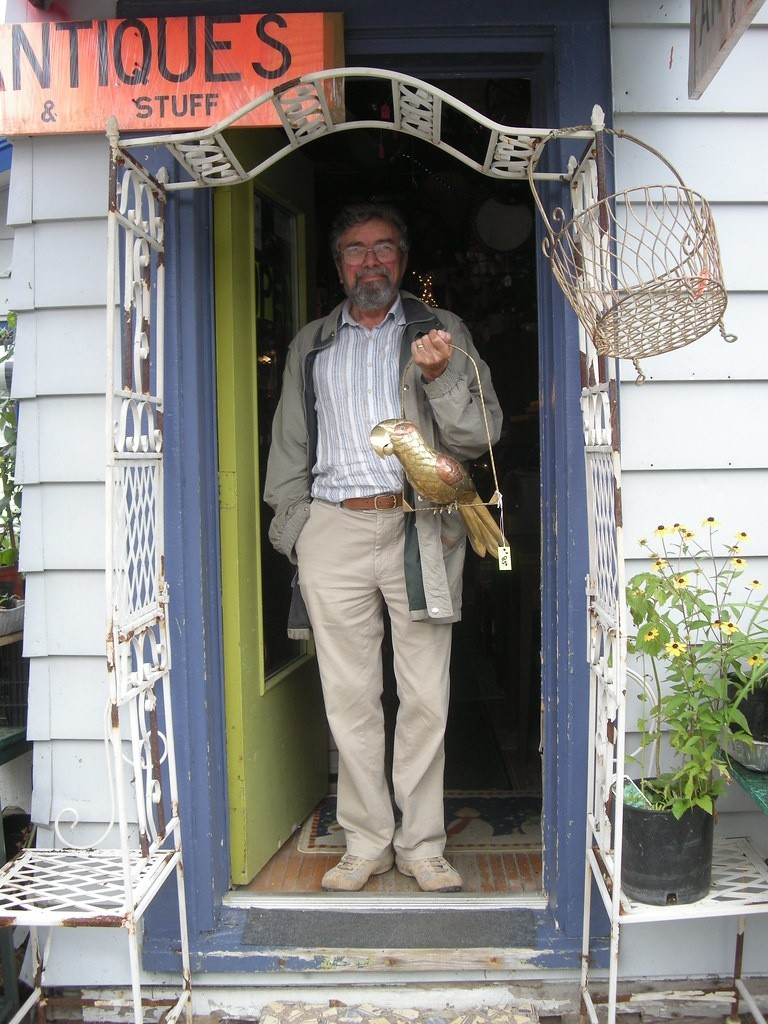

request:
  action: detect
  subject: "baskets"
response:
[529,125,738,390]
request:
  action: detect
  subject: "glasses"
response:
[339,243,400,265]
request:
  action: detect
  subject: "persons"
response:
[261,198,502,896]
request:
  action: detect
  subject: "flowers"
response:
[616,518,768,820]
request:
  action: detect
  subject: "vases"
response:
[611,779,716,907]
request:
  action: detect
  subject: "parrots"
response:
[370,419,514,567]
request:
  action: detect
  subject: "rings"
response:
[417,344,424,348]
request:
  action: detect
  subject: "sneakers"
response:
[395,854,462,891]
[321,849,394,890]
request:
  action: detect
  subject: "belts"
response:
[342,494,401,509]
